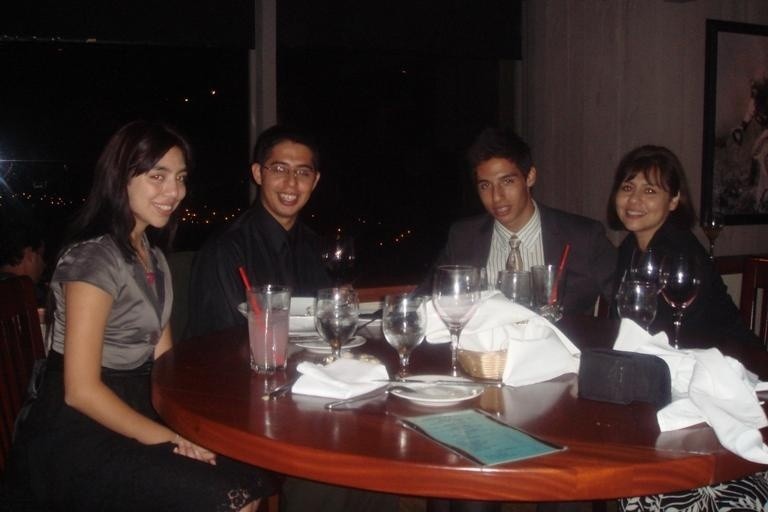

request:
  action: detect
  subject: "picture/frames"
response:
[700,17,768,226]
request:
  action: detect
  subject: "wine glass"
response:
[433,265,483,386]
[495,270,536,314]
[700,211,725,264]
[381,291,427,381]
[314,289,359,361]
[617,243,700,351]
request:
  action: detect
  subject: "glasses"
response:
[263,164,314,181]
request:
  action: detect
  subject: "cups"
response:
[247,286,290,372]
[533,265,567,323]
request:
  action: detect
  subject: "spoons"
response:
[326,386,417,409]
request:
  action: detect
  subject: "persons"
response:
[1,225,51,324]
[1,116,266,512]
[187,119,332,335]
[411,130,619,317]
[602,143,768,512]
[730,68,768,185]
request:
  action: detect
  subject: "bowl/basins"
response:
[352,298,422,340]
[237,295,353,341]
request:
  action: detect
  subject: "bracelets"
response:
[174,434,179,443]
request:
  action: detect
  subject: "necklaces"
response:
[136,246,155,285]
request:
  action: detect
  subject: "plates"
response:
[390,371,485,407]
[296,334,367,352]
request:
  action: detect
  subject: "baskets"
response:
[459,349,507,381]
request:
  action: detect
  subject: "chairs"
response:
[713,256,768,346]
[0,275,48,466]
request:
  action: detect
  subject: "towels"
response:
[291,357,389,400]
[610,318,768,467]
[418,291,582,387]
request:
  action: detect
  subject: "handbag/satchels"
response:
[578,347,671,407]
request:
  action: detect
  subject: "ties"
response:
[506,235,523,272]
